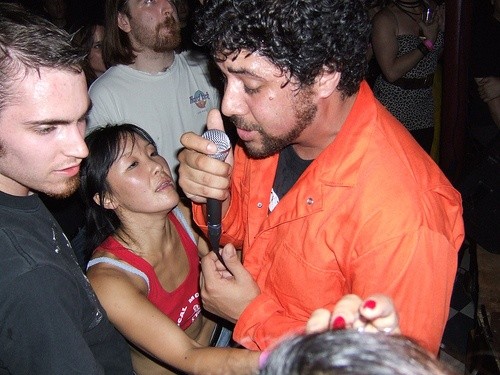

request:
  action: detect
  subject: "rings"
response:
[435,14,439,16]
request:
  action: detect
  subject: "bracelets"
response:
[417,39,433,56]
[258,348,270,371]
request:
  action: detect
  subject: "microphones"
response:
[200,129,231,253]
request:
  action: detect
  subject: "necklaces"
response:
[393,0,427,23]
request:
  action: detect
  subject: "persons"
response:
[178,0,465,357]
[463,0,500,254]
[0,19,132,375]
[260,293,456,375]
[80,122,271,375]
[86,1,237,203]
[41,0,106,86]
[370,0,445,156]
